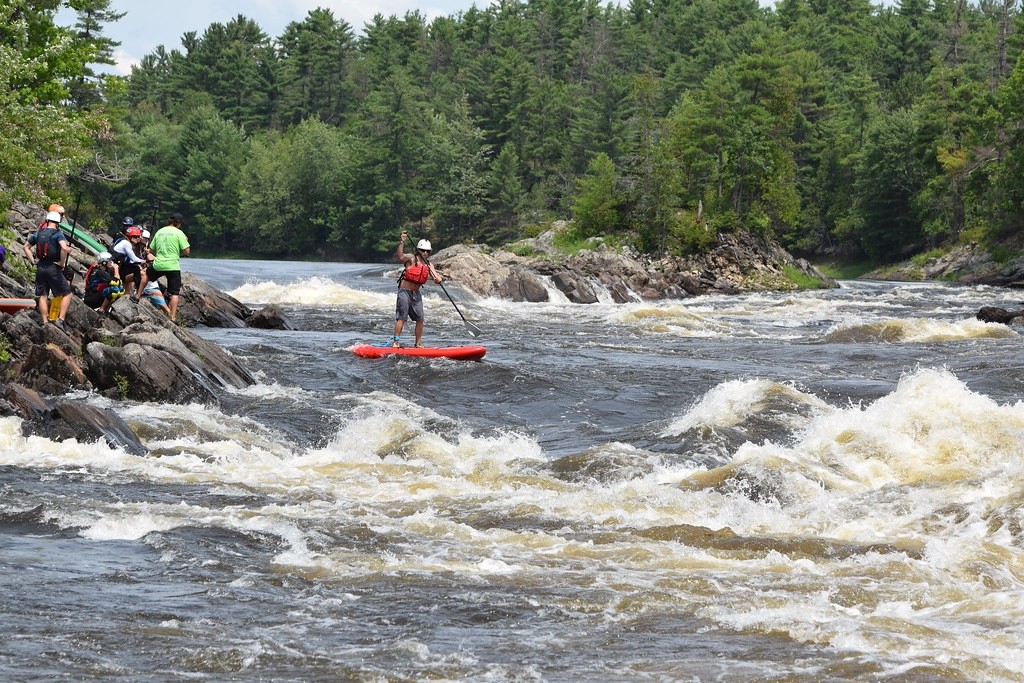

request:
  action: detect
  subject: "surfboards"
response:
[353,344,486,360]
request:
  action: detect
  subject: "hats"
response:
[169,213,184,221]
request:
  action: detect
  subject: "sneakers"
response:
[94,307,106,317]
[55,318,70,335]
[129,295,140,304]
[172,320,180,327]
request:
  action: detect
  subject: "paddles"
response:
[49,190,84,321]
[404,230,481,338]
[144,206,160,259]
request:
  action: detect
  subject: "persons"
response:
[36,204,73,324]
[108,216,171,317]
[23,211,73,337]
[84,252,125,319]
[392,230,442,348]
[130,213,190,327]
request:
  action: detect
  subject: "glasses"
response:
[131,234,140,239]
[60,212,64,217]
[418,248,433,254]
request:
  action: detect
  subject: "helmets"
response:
[125,227,141,237]
[46,211,62,223]
[97,251,112,267]
[129,224,150,238]
[417,239,434,251]
[123,217,133,225]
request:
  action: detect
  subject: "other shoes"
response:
[392,342,400,347]
[415,342,423,348]
